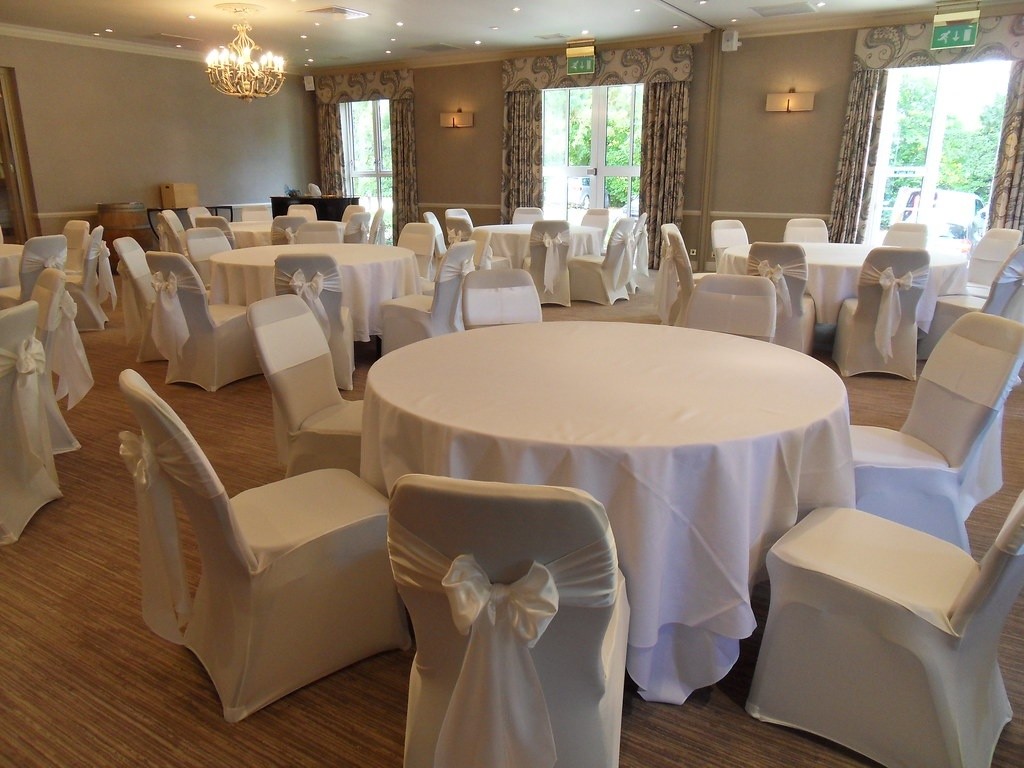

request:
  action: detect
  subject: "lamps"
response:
[765,90,814,113]
[204,3,286,103]
[439,109,474,127]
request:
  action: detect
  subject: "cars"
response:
[568,176,611,210]
[618,193,641,227]
[892,185,987,239]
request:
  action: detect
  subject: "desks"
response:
[468,222,603,267]
[227,221,345,248]
[359,319,855,706]
[0,244,24,289]
[716,243,967,335]
[147,206,233,238]
[271,196,358,219]
[209,243,420,341]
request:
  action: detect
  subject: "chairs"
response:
[0,204,1024,768]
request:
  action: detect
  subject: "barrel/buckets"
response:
[98,203,146,275]
